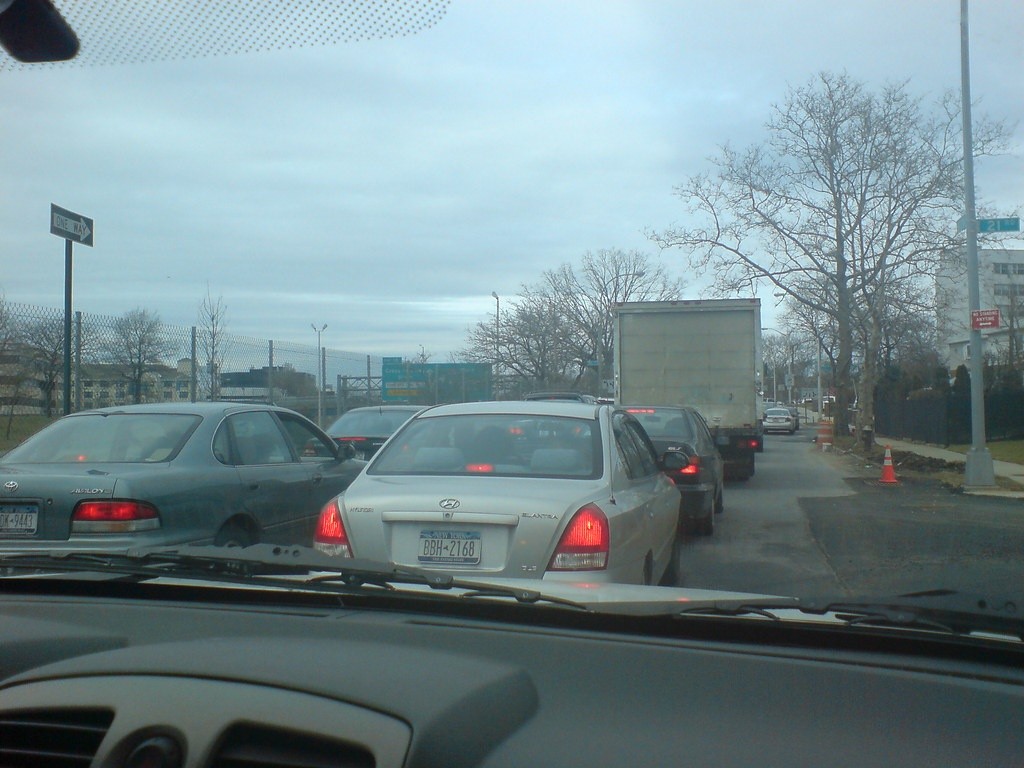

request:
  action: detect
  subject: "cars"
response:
[506,390,615,464]
[576,406,729,534]
[763,407,797,436]
[303,403,447,471]
[785,407,801,430]
[309,396,691,589]
[0,398,378,578]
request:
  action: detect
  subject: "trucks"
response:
[607,297,767,480]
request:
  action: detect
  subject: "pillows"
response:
[530,449,586,474]
[413,447,463,470]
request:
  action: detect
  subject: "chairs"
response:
[559,433,594,468]
[371,417,393,435]
[222,435,257,464]
[465,424,518,465]
[663,417,688,438]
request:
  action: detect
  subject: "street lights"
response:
[760,326,803,403]
[598,270,645,395]
[310,322,328,429]
[490,289,502,401]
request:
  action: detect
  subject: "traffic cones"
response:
[877,444,902,483]
